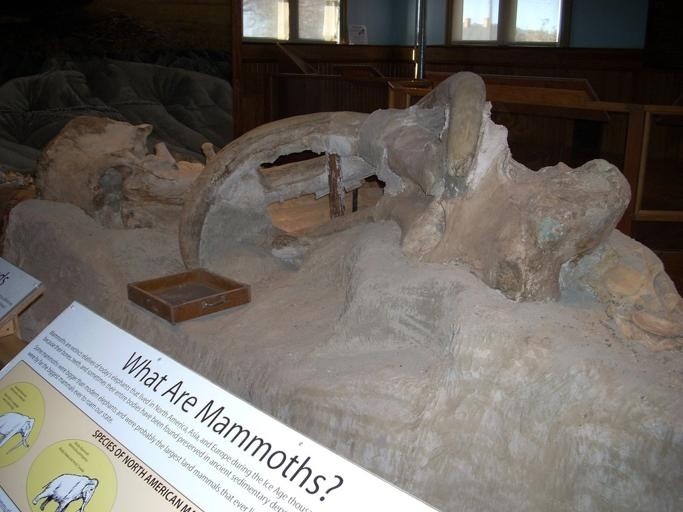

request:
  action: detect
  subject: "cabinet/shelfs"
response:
[236,58,408,140]
[388,75,683,298]
